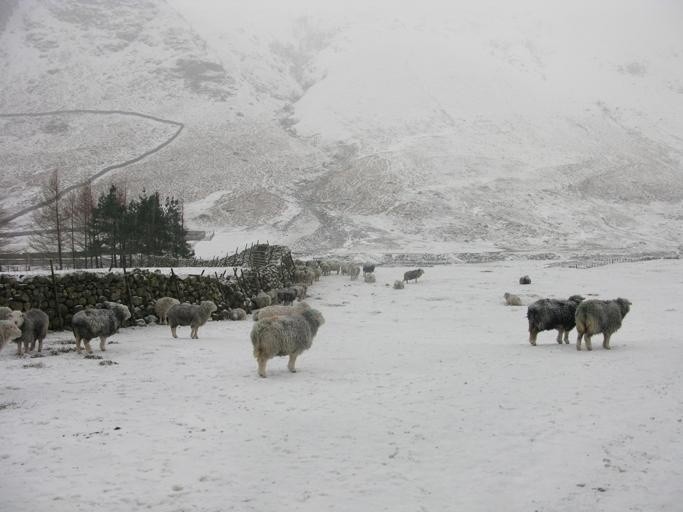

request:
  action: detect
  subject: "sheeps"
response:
[250,299,327,379]
[504,289,526,308]
[527,292,633,352]
[402,268,424,284]
[0,304,50,354]
[393,279,404,290]
[293,258,377,285]
[70,299,132,354]
[520,274,532,285]
[153,294,218,338]
